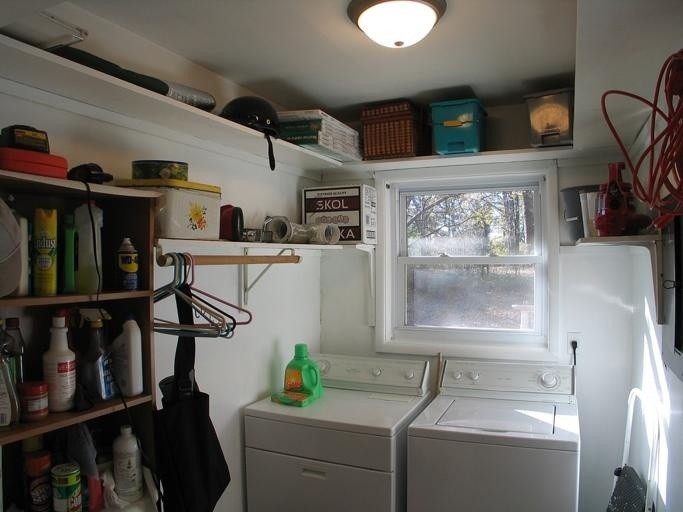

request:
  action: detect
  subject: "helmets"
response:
[221,96,280,138]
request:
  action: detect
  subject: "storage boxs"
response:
[521,88,573,147]
[112,179,222,240]
[358,100,420,161]
[302,183,379,245]
[430,97,489,155]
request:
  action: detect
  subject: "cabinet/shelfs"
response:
[1,169,164,511]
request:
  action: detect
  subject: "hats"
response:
[0,198,21,297]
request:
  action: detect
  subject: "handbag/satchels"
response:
[156,284,229,511]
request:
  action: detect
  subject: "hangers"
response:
[148,252,253,339]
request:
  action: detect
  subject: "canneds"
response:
[15,380,49,422]
[51,463,83,511]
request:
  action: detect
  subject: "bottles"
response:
[0,318,76,430]
[112,426,144,503]
[117,238,138,290]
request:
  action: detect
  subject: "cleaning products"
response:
[281,342,321,399]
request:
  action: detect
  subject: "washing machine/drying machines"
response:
[243,350,429,512]
[405,355,582,512]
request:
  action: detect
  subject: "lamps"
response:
[348,1,448,49]
